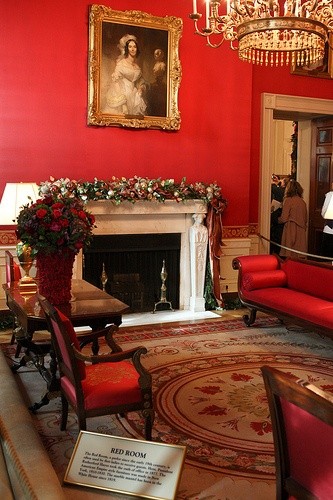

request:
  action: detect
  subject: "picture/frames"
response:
[86,3,183,131]
[290,32,333,80]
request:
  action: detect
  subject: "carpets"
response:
[0,316,333,500]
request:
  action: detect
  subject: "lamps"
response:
[0,183,41,283]
[189,0,333,67]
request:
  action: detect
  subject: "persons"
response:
[270,173,294,253]
[277,181,307,260]
[321,182,333,261]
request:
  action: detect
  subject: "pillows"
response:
[244,270,287,291]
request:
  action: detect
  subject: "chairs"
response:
[260,365,333,500]
[37,293,154,440]
[5,251,22,358]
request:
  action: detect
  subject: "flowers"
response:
[13,175,221,253]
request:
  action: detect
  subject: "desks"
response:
[2,279,130,411]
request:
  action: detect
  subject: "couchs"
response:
[233,254,333,338]
